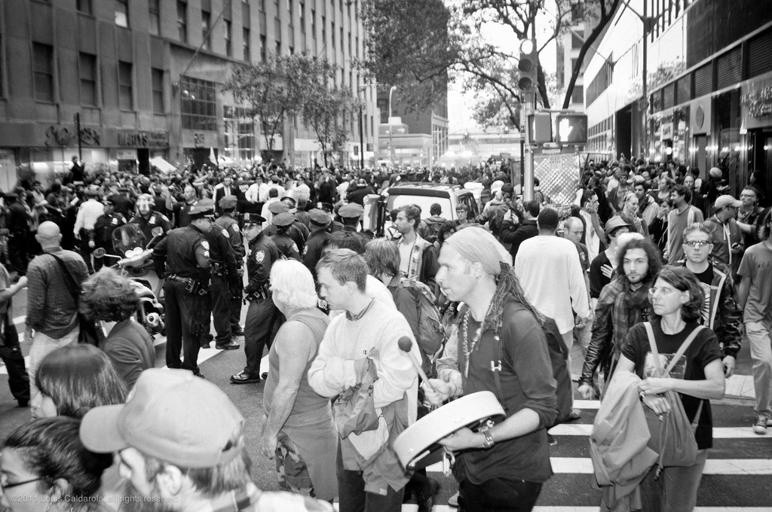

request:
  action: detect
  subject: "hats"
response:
[78,367,246,469]
[87,191,99,196]
[100,195,363,229]
[604,215,632,234]
[714,194,743,209]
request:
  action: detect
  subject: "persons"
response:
[612,266,726,511]
[307,247,424,512]
[1,416,114,512]
[260,258,339,507]
[435,226,559,511]
[80,366,336,512]
[1,158,771,435]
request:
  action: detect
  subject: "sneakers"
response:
[752,411,772,434]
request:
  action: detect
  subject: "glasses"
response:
[0,476,43,495]
[684,240,710,246]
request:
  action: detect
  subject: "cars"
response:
[380,182,480,234]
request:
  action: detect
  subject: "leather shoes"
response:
[197,327,269,384]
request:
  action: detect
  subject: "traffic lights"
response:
[556,114,591,145]
[516,38,539,94]
[528,111,552,145]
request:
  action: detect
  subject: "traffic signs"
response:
[387,84,400,167]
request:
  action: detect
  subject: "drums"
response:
[392,390,506,473]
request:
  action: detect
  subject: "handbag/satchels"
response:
[78,319,105,346]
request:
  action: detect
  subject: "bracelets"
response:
[476,425,495,448]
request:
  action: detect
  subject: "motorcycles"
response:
[94,226,167,338]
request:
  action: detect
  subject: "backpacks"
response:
[639,322,707,468]
[413,280,446,355]
[501,301,573,426]
